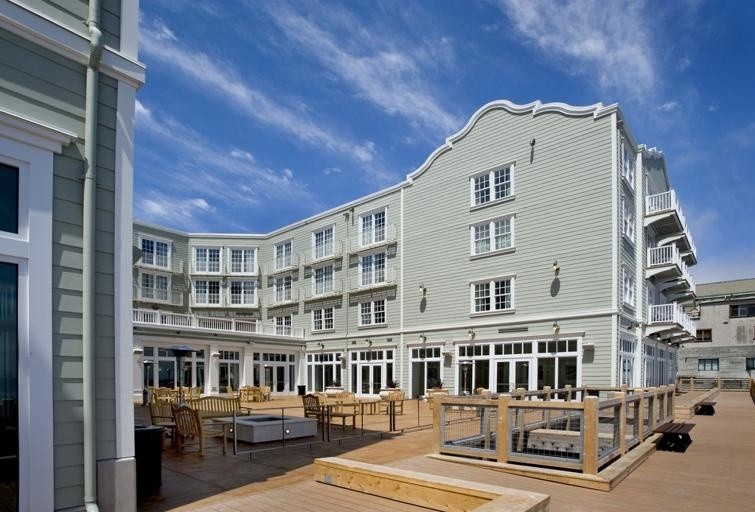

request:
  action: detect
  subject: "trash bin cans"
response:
[589,390,598,395]
[135,426,163,497]
[298,386,305,395]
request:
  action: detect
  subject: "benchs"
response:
[652,422,696,452]
[148,386,253,456]
[301,387,405,430]
[426,388,450,409]
[697,402,717,415]
[226,385,272,402]
